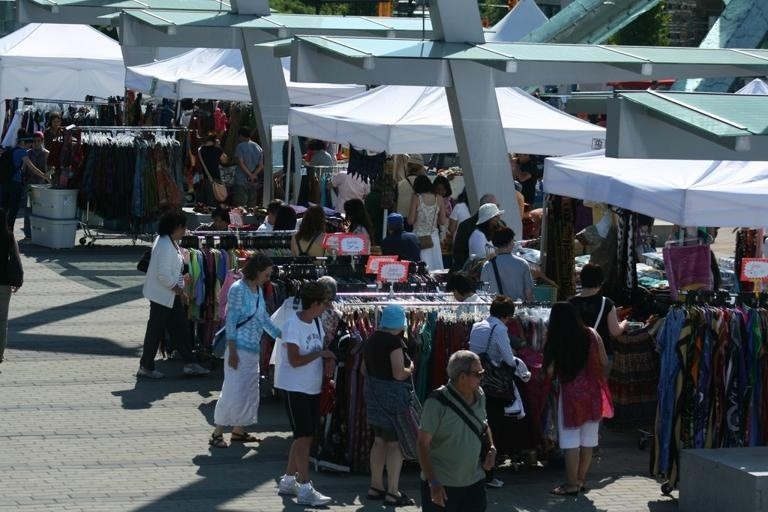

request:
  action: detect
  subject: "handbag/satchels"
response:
[213,325,226,357]
[418,236,433,250]
[477,351,516,408]
[212,183,228,201]
[481,433,495,476]
[320,376,336,414]
[136,252,151,273]
[393,391,423,461]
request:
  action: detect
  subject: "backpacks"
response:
[0,146,25,177]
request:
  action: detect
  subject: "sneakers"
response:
[488,478,503,488]
[136,368,164,379]
[278,477,297,495]
[184,364,211,376]
[298,485,331,506]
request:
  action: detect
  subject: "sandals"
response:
[367,486,386,499]
[384,490,415,507]
[209,432,227,448]
[551,484,578,495]
[230,430,260,442]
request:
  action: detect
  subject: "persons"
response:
[137,126,630,512]
[0,130,51,232]
[0,208,22,362]
[24,131,50,240]
[44,114,64,166]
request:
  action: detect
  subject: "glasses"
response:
[470,369,485,377]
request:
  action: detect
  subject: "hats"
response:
[16,131,43,141]
[476,203,504,226]
[408,154,424,166]
[387,212,404,224]
[381,305,405,329]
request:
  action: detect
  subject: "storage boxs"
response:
[31,186,80,219]
[30,216,79,250]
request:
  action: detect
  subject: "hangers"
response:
[266,264,350,278]
[334,293,550,337]
[81,128,180,148]
[204,233,291,248]
